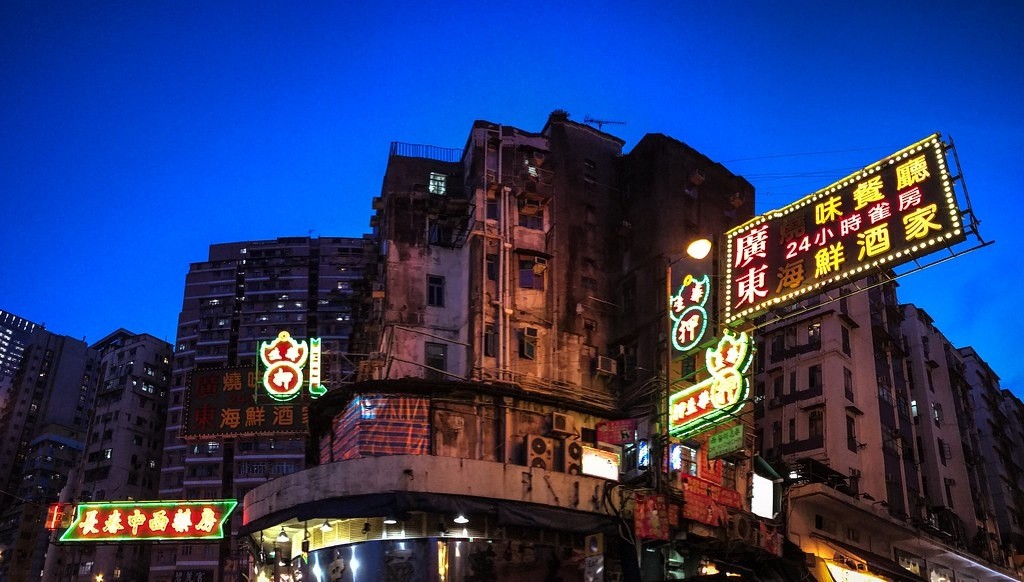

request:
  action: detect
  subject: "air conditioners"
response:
[611,345,630,357]
[594,355,617,377]
[560,438,593,476]
[584,532,608,557]
[788,326,797,336]
[523,433,555,470]
[549,412,574,436]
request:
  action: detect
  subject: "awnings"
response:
[813,531,930,582]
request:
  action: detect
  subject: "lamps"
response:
[872,499,891,508]
[276,526,290,543]
[854,492,875,501]
[383,517,398,525]
[454,514,469,523]
[319,519,333,532]
